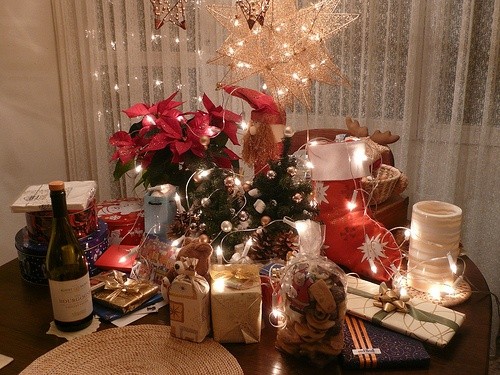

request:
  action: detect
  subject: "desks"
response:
[0,245,492,375]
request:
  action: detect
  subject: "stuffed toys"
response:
[159,236,213,301]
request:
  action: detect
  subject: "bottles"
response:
[46,181,94,333]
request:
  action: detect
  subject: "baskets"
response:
[361,163,401,207]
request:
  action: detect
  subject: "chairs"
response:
[290,129,394,168]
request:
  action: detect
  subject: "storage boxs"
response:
[210,265,262,344]
[144,195,175,234]
[373,195,409,231]
